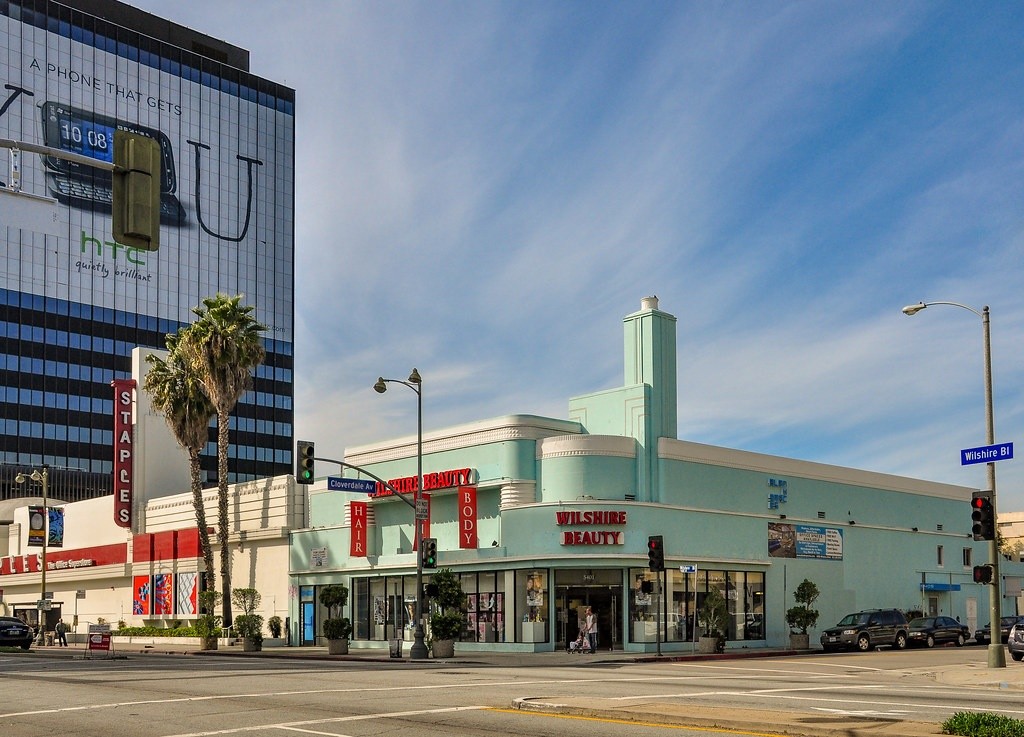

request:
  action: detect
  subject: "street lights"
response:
[900,300,1006,669]
[15,467,47,648]
[372,367,428,660]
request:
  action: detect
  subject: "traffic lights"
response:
[972,566,991,583]
[297,441,315,485]
[971,490,994,541]
[648,535,664,572]
[424,537,437,568]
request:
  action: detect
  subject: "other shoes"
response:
[589,651,595,654]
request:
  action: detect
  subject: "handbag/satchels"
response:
[583,637,591,649]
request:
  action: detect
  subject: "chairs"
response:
[1001,619,1009,625]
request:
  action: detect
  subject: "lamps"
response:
[912,527,918,532]
[849,520,855,525]
[238,542,243,551]
[492,540,499,547]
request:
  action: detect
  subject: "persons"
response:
[575,608,597,653]
[528,579,539,601]
[55,618,68,647]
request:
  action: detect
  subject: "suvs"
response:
[820,608,909,652]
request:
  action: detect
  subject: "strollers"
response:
[566,629,592,654]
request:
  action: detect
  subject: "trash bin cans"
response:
[44,631,56,646]
[389,637,403,658]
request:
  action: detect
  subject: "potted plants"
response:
[784,579,820,650]
[697,586,728,654]
[195,591,225,651]
[426,567,466,657]
[230,588,264,652]
[318,586,353,655]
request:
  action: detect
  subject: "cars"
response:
[1008,619,1024,661]
[0,616,34,651]
[907,616,971,648]
[975,616,1024,645]
